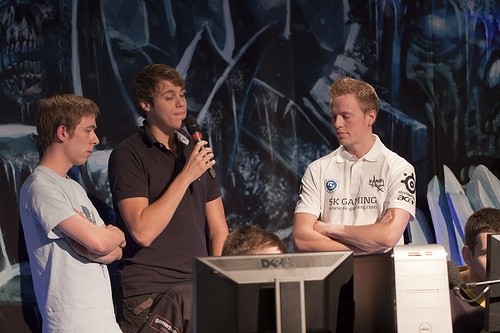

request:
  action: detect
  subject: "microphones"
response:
[446,261,461,294]
[184,118,216,179]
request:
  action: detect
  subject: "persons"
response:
[450,206,500,333]
[221,224,287,257]
[108,63,229,333]
[292,74,416,254]
[19,94,126,333]
